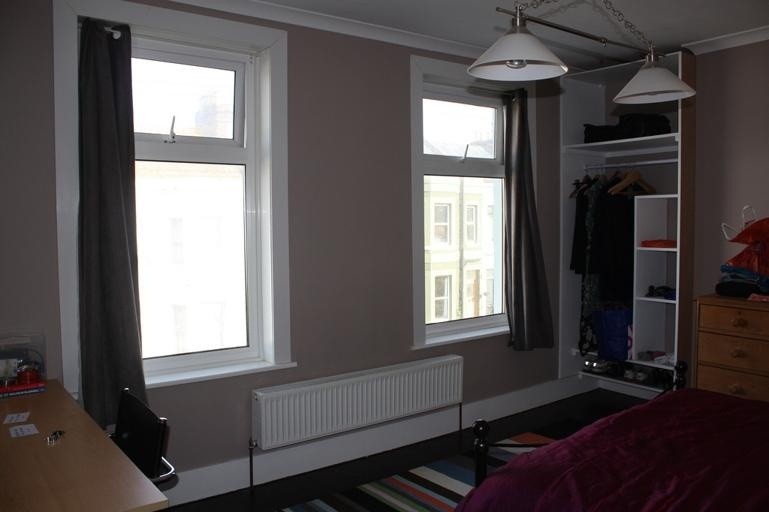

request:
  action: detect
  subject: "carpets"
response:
[279,431,560,512]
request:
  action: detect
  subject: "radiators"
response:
[251,354,464,451]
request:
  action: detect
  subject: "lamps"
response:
[467,7,698,107]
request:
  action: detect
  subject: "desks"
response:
[0,379,168,512]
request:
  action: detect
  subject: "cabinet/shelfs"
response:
[695,295,769,402]
[557,51,698,394]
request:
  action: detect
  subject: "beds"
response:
[453,359,769,511]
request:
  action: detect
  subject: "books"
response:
[0,378,48,398]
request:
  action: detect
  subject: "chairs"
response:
[107,387,174,487]
